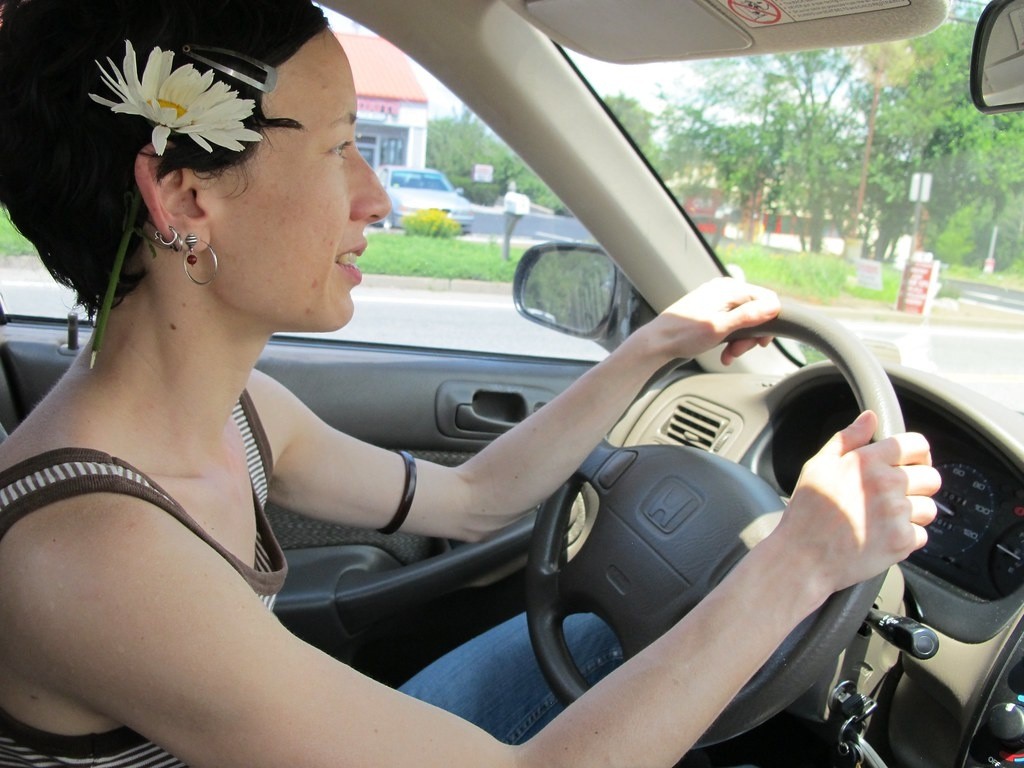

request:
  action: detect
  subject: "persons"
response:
[0,0,942,768]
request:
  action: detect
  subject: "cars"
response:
[372,165,473,237]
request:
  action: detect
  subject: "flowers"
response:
[86,33,261,373]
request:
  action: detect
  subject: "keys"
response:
[838,692,877,725]
[832,741,856,768]
